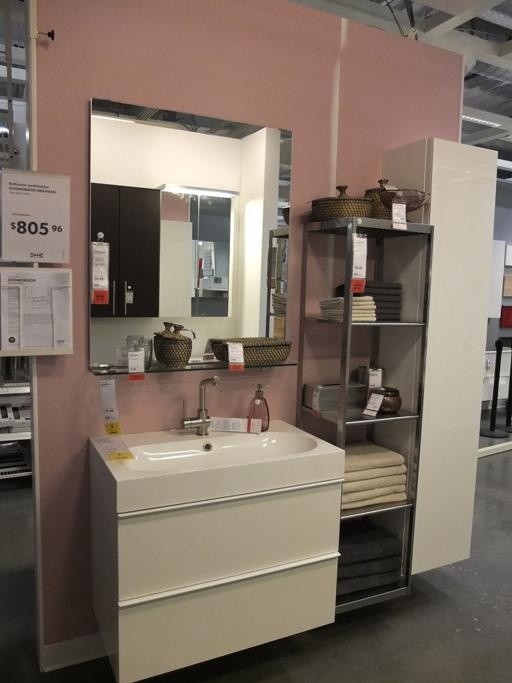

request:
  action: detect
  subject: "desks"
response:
[480,337,511,438]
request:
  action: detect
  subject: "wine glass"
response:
[378,188,431,224]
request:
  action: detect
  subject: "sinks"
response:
[121,432,317,470]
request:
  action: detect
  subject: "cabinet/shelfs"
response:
[90,182,161,319]
[296,211,435,632]
[0,0,41,482]
[268,227,289,340]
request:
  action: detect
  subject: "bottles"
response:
[126,285,133,304]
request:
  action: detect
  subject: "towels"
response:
[339,443,407,509]
[319,279,402,322]
[337,521,400,598]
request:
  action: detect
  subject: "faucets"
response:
[181,375,220,436]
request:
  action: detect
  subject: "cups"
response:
[133,337,153,370]
[125,335,143,355]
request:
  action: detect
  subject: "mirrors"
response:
[157,191,241,319]
[84,95,294,376]
